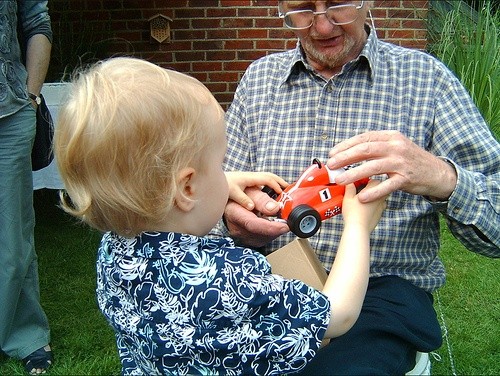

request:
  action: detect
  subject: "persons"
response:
[54,58,389,376]
[0,0,53,375]
[206,0,500,376]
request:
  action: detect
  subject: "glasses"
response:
[277,0,364,30]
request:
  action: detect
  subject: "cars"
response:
[262,156,371,238]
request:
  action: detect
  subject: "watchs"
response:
[28,92,42,105]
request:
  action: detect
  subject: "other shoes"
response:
[23,344,54,375]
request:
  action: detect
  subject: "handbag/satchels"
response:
[32,93,55,172]
